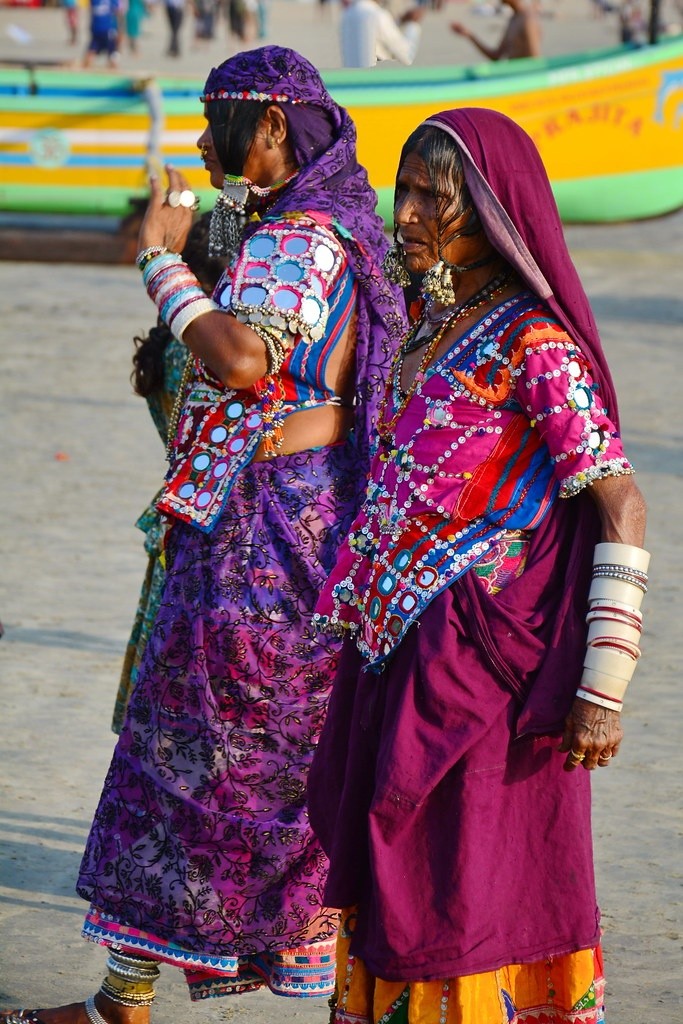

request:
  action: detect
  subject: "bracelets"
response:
[576,540,649,712]
[135,245,218,344]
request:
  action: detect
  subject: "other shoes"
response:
[0,1001,154,1024]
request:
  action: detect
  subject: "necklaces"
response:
[376,269,515,440]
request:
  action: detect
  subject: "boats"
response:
[1,32,683,230]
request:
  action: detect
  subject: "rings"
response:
[600,753,613,760]
[165,190,200,212]
[570,747,587,767]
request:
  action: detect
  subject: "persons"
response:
[310,107,651,1024]
[59,0,641,68]
[0,43,409,1024]
